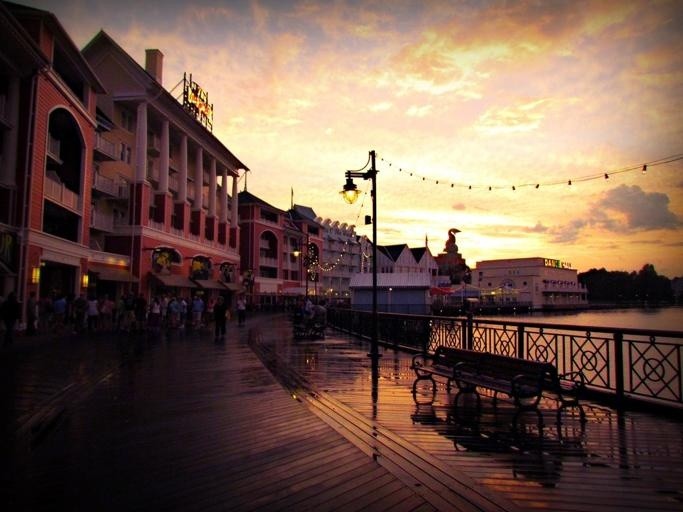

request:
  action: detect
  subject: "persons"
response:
[0,282,249,342]
[279,291,347,314]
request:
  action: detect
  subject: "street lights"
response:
[291,232,314,299]
[462,267,471,307]
[341,147,379,400]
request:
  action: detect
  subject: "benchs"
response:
[408,347,550,433]
[312,316,327,339]
[411,404,558,489]
[545,365,590,425]
[290,312,312,338]
[472,417,592,467]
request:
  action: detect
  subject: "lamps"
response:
[27,262,45,286]
[81,271,89,289]
